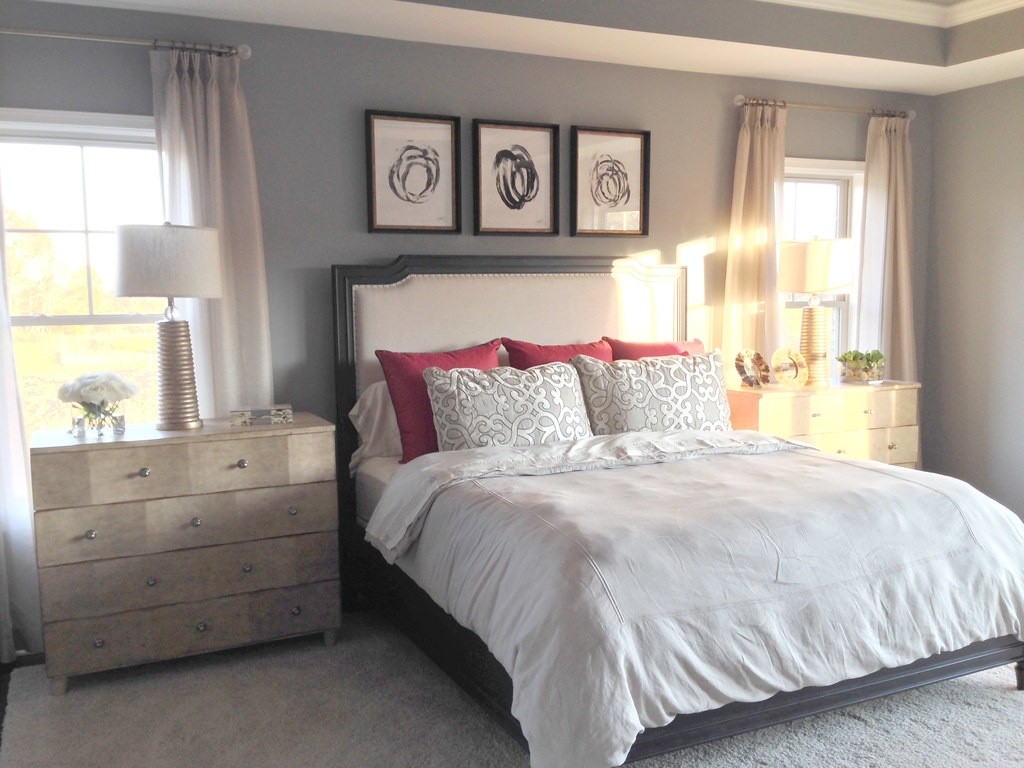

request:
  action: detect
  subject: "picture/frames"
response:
[570,125,649,236]
[364,109,461,234]
[471,118,561,235]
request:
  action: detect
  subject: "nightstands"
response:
[30,410,344,688]
[725,380,922,472]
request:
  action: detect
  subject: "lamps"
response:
[115,221,226,431]
[783,235,852,391]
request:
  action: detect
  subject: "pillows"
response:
[422,362,593,453]
[603,335,702,361]
[498,336,614,371]
[373,338,500,464]
[571,353,733,438]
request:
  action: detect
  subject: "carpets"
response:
[0,622,1024,766]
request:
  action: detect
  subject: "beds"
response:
[331,254,1023,768]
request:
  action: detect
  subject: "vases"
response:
[72,415,125,437]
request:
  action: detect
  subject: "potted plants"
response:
[836,350,885,384]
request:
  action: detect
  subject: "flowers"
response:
[57,371,137,431]
